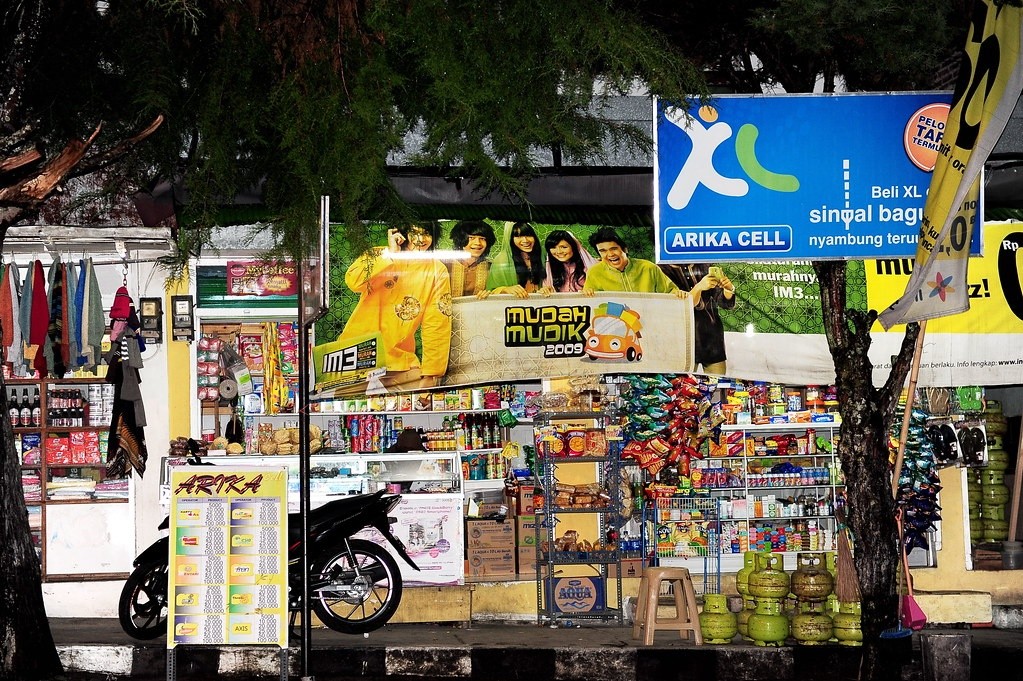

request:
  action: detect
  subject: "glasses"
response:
[406,230,429,236]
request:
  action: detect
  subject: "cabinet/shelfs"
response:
[532,411,624,627]
[5,378,136,583]
[658,421,934,576]
[186,257,550,491]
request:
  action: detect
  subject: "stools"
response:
[633,567,702,646]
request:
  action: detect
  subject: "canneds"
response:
[327,415,404,454]
[87,383,115,427]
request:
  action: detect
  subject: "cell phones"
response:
[709,267,722,278]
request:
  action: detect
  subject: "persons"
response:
[337,221,452,390]
[655,263,736,376]
[537,230,601,298]
[581,229,689,299]
[486,222,547,300]
[437,220,496,301]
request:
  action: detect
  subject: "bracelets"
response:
[420,375,437,383]
[724,285,735,295]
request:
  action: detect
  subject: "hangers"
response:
[9,249,91,260]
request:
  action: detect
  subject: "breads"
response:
[210,436,244,454]
[259,425,322,454]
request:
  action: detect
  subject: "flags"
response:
[876,0,1023,332]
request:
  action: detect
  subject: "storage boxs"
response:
[320,389,501,413]
[544,564,605,613]
[465,485,545,575]
[63,365,109,378]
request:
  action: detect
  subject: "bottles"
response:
[10,388,83,427]
[404,410,500,451]
[607,527,649,558]
[630,482,643,509]
[746,469,829,486]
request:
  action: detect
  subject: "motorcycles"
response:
[116,438,420,645]
